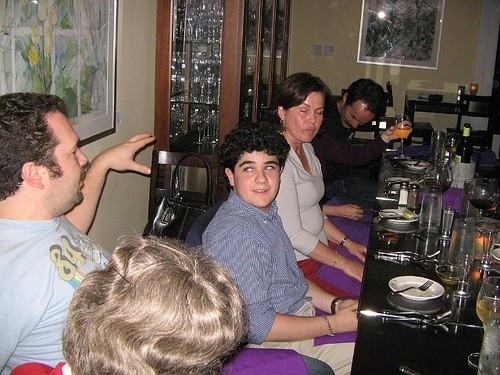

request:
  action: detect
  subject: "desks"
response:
[350,149,500,375]
[403,89,500,147]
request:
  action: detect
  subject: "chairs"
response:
[386,80,434,149]
[148,149,229,222]
[185,199,226,251]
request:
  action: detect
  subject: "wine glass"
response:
[468,178,497,217]
[171,0,284,147]
[394,115,411,159]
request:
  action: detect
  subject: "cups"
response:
[471,83,478,95]
[420,128,500,375]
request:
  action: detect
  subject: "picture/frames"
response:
[0,0,116,149]
[356,0,445,71]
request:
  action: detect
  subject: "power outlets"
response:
[322,45,335,56]
[312,44,322,55]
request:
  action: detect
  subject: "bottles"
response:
[398,181,420,209]
[243,82,253,123]
[454,123,472,163]
[457,85,465,103]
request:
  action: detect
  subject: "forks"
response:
[392,280,433,294]
[379,249,440,258]
[383,309,451,320]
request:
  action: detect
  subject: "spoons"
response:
[381,316,452,317]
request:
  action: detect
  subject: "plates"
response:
[388,274,446,302]
[386,177,412,188]
[379,209,420,224]
[401,161,430,170]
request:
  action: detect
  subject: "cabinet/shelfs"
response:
[155,0,291,188]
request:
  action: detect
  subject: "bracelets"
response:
[340,236,349,245]
[331,298,340,314]
[331,250,338,266]
[321,315,333,336]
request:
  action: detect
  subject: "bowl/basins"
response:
[435,264,468,283]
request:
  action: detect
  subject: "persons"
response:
[0,92,156,375]
[12,236,249,375]
[201,123,359,375]
[270,73,367,297]
[322,204,363,220]
[311,78,412,209]
